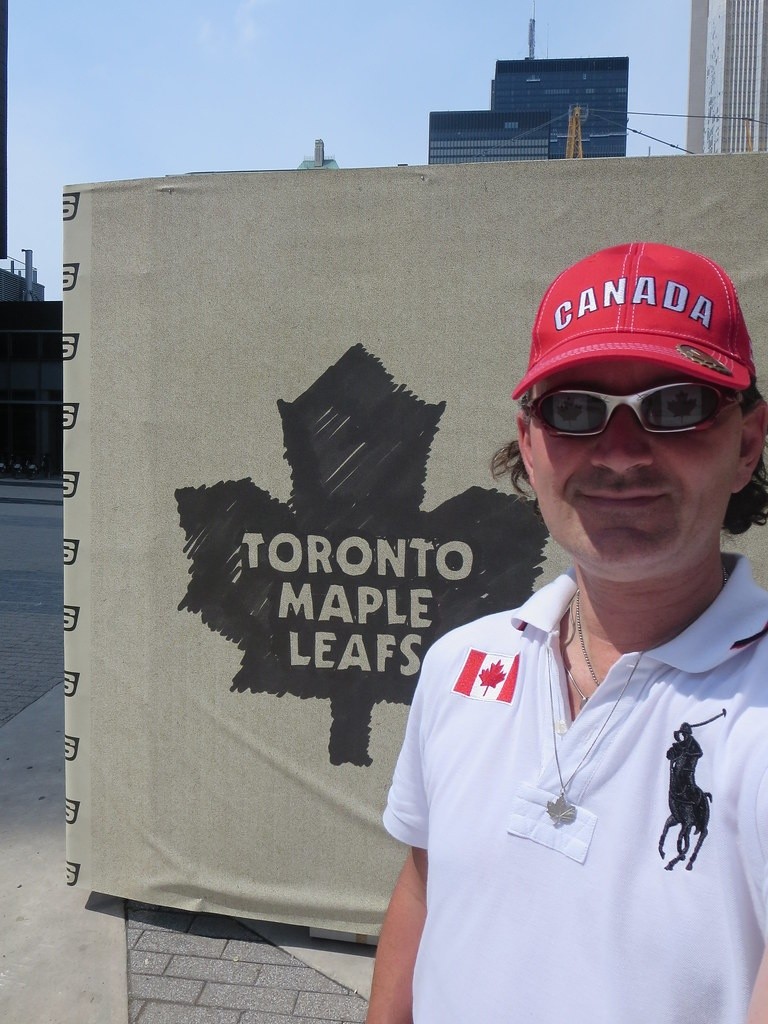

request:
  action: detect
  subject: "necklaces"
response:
[546,566,726,828]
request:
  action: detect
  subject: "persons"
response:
[365,242,767,1024]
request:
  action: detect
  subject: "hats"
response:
[512,245,756,395]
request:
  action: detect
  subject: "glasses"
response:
[526,382,746,436]
